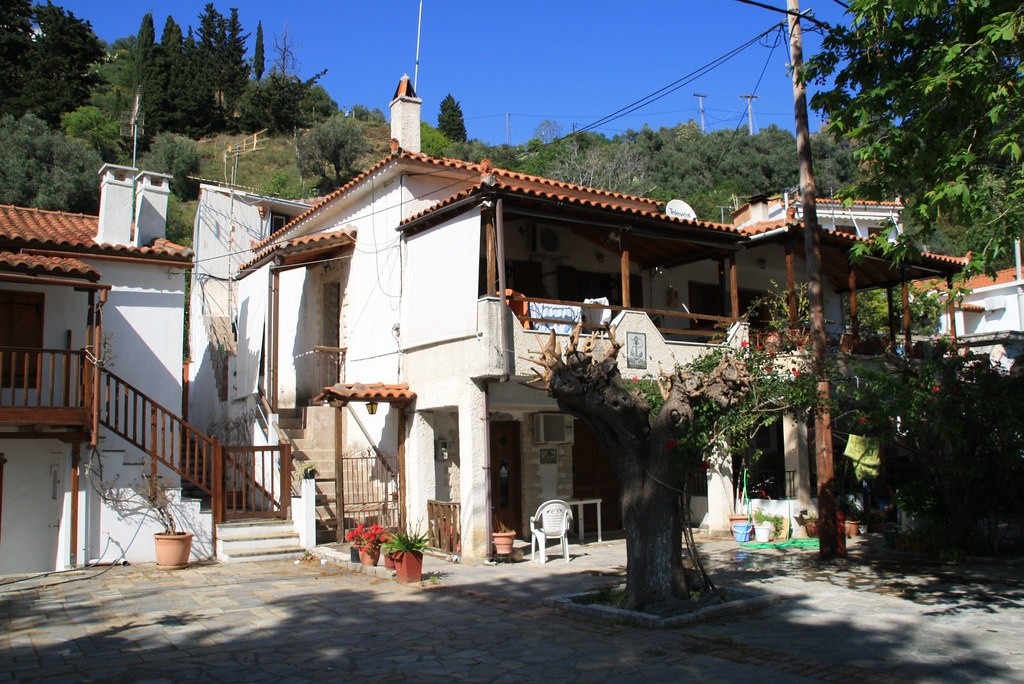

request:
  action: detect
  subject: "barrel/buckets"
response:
[754,526,770,543]
[732,523,752,542]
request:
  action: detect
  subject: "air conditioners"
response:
[533,413,574,445]
[528,223,573,257]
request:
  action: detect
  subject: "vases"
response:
[492,532,517,554]
[359,546,380,566]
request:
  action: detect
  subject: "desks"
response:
[566,497,602,542]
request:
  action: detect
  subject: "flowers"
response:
[346,523,390,553]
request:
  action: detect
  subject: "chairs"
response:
[530,500,573,564]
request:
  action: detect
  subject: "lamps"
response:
[366,402,378,415]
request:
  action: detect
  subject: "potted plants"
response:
[140,473,194,566]
[382,518,438,584]
[701,415,947,554]
[295,464,320,485]
